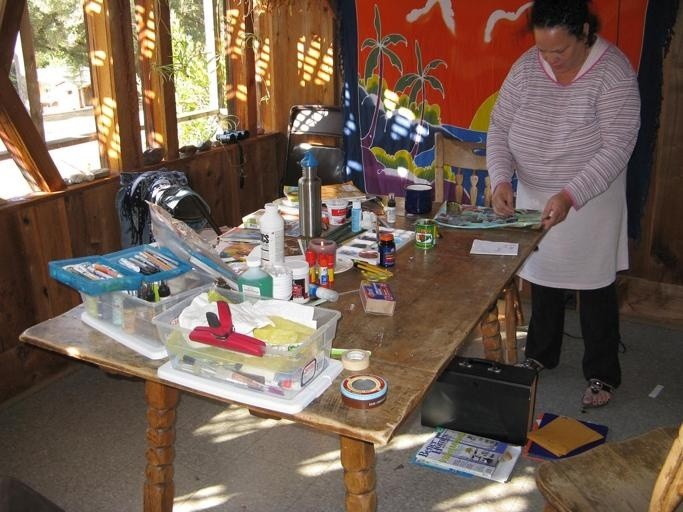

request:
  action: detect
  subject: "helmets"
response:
[283,254,354,275]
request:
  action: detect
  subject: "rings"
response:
[118,248,179,275]
[66,263,125,281]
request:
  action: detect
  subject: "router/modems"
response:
[341,348,370,371]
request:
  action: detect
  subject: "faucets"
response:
[580,377,615,409]
[512,357,546,371]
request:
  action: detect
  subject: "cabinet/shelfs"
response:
[259,202,284,267]
[236,257,272,301]
[350,200,362,233]
[82,277,171,335]
[297,151,323,238]
[386,193,396,223]
[378,234,395,267]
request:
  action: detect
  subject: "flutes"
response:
[47,244,193,296]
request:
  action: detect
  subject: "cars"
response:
[305,237,336,283]
[325,199,349,225]
[404,184,432,214]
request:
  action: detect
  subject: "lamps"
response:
[429,132,528,367]
[277,101,348,194]
[533,422,682,512]
[173,193,231,244]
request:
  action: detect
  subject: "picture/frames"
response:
[18,184,552,512]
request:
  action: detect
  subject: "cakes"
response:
[414,219,438,249]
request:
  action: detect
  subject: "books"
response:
[77,268,219,362]
[148,285,344,417]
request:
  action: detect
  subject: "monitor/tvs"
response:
[416,426,508,478]
[244,180,366,231]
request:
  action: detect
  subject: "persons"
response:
[485,0,643,409]
